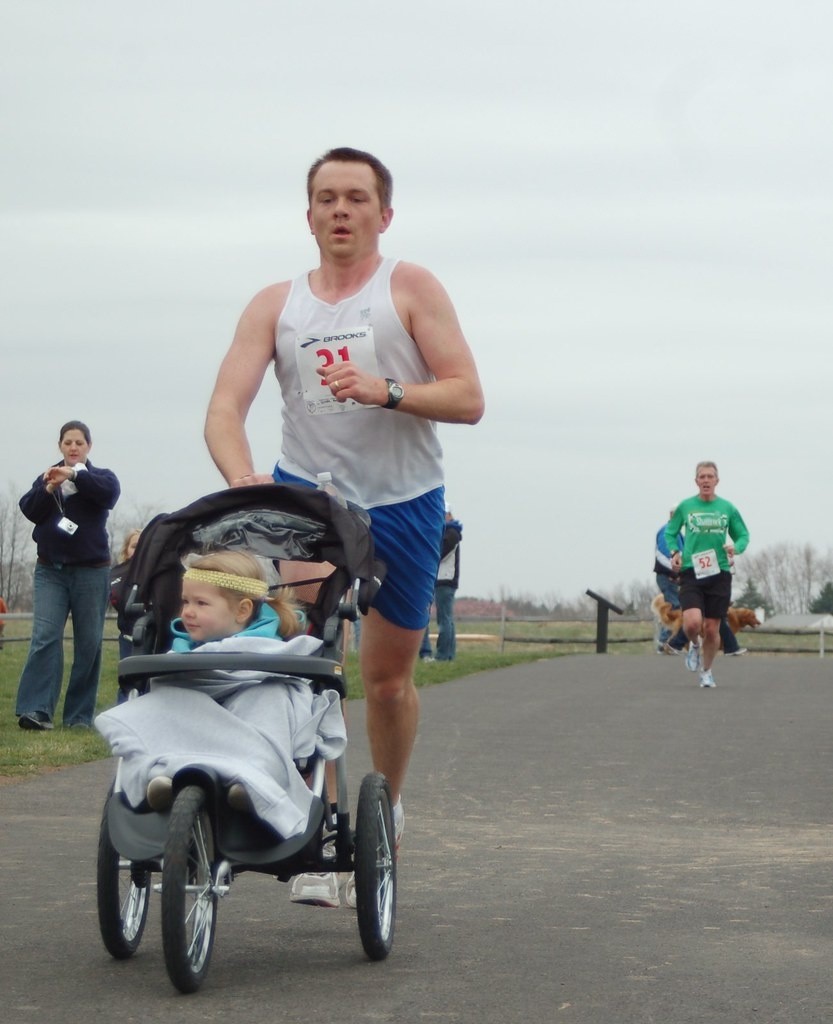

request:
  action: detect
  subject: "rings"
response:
[334,380,342,392]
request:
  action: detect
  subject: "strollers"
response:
[91,482,399,994]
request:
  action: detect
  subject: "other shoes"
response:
[70,723,90,730]
[18,712,54,730]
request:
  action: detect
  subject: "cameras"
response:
[58,517,78,535]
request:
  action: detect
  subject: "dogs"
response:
[649,595,762,658]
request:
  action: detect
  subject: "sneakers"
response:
[290,814,340,908]
[686,641,700,672]
[668,643,687,655]
[346,810,404,908]
[700,671,716,688]
[725,647,747,656]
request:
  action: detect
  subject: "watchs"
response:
[383,376,405,409]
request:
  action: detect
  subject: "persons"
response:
[116,530,142,565]
[13,420,120,733]
[351,500,462,663]
[0,596,8,651]
[653,508,748,659]
[664,461,750,686]
[202,146,487,909]
[145,551,305,815]
